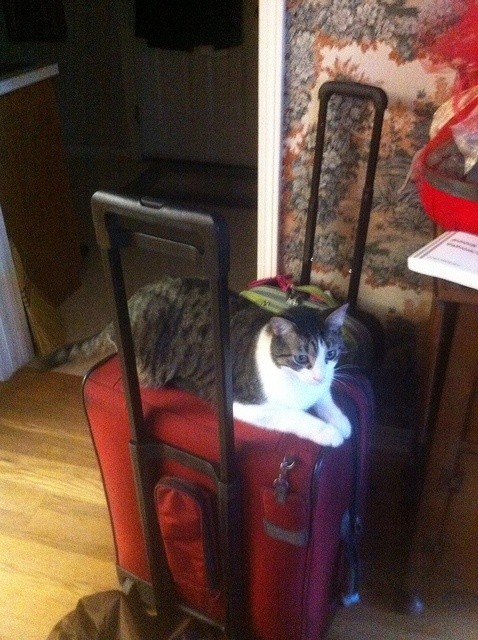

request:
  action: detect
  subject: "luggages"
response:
[239,80,388,392]
[81,190,376,640]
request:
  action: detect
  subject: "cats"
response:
[35,274,352,449]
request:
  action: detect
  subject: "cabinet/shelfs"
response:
[392,274,477,595]
[0,59,86,359]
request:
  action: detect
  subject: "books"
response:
[406,229,478,290]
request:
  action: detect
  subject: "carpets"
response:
[49,192,257,378]
